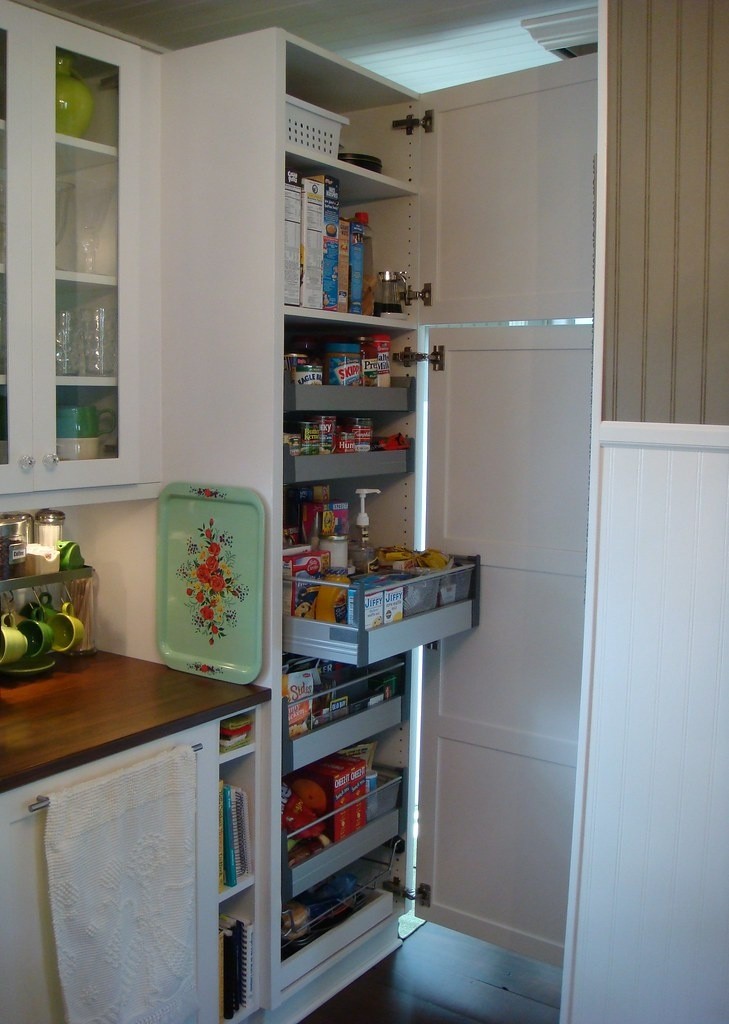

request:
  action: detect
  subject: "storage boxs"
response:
[283,174,404,843]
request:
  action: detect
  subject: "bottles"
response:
[0,509,66,580]
[315,567,351,624]
[293,333,391,387]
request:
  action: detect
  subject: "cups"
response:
[0,592,85,665]
[373,270,409,317]
[56,405,116,460]
[57,304,118,377]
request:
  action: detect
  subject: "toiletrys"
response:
[347,487,381,577]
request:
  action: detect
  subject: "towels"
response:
[219,711,256,755]
[35,743,202,1024]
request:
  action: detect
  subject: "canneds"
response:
[315,568,351,624]
[347,543,374,574]
[287,333,391,388]
[318,534,349,568]
[284,415,373,455]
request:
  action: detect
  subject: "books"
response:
[219,913,256,1024]
[218,778,253,894]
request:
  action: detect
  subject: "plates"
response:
[0,655,56,674]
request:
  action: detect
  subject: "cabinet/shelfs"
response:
[0,1,161,495]
[158,28,600,1013]
[0,706,259,1024]
